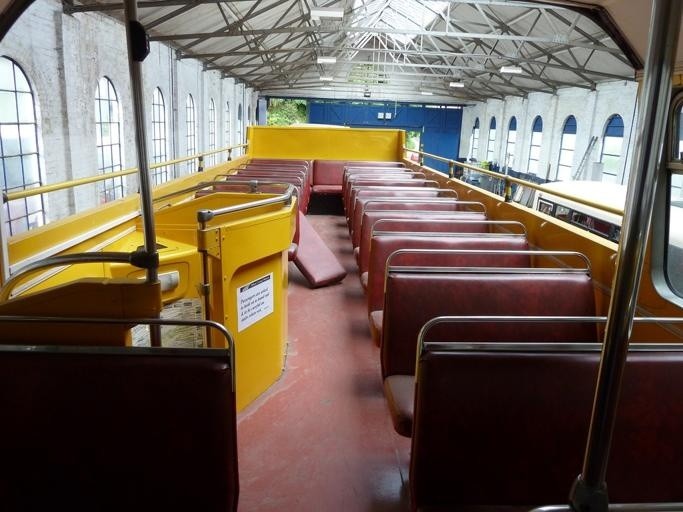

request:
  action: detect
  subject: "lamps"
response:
[308,6,345,19]
[317,54,336,64]
[500,66,523,74]
[450,81,465,88]
